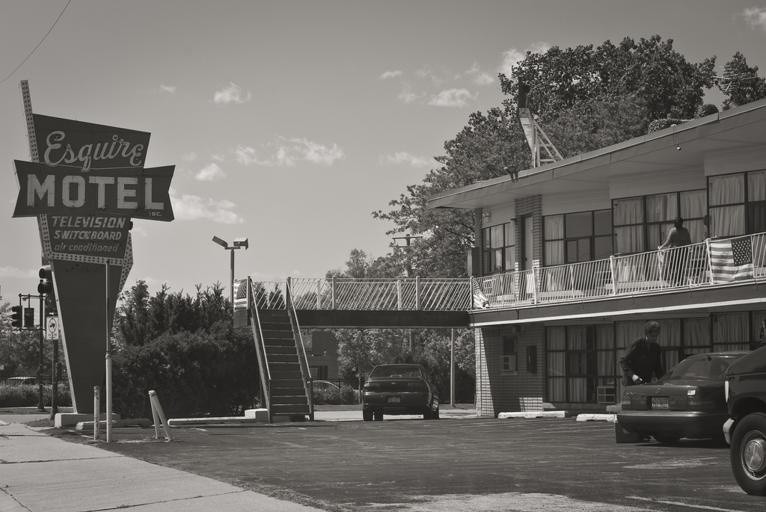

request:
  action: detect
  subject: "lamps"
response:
[484,208,493,223]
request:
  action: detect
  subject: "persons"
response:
[618,321,666,385]
[659,216,691,287]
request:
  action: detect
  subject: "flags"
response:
[705,239,754,284]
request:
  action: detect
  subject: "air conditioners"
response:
[483,279,501,297]
[501,354,517,372]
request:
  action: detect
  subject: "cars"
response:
[615,351,750,446]
[307,379,338,392]
[723,344,766,497]
[361,363,440,420]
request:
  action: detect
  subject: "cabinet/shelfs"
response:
[597,385,616,406]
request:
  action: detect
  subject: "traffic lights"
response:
[36,266,53,296]
[10,305,18,326]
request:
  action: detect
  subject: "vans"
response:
[7,377,37,388]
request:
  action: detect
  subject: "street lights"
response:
[214,234,249,309]
